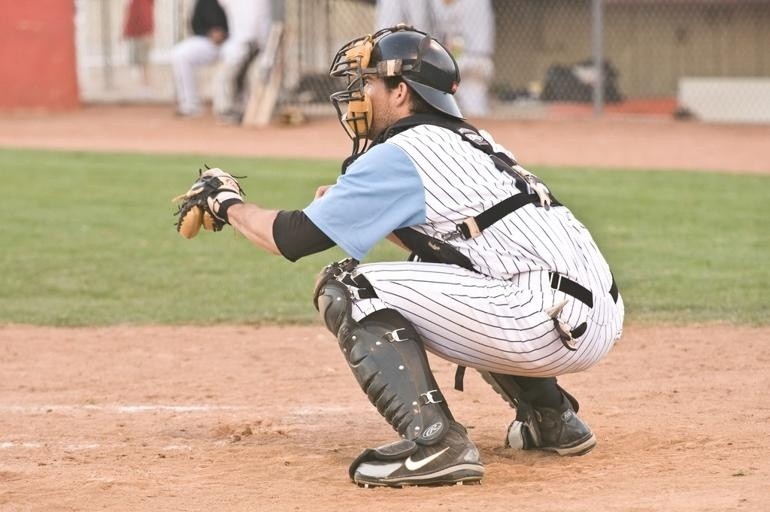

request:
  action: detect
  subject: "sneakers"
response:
[506,390,597,458]
[353,419,486,489]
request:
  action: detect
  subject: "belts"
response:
[549,271,619,309]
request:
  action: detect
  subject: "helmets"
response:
[346,23,466,140]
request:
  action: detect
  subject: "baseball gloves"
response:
[172,165,247,240]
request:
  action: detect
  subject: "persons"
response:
[182,22,629,491]
[168,1,284,127]
[373,1,435,38]
[427,0,499,118]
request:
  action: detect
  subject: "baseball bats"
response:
[241,17,282,123]
